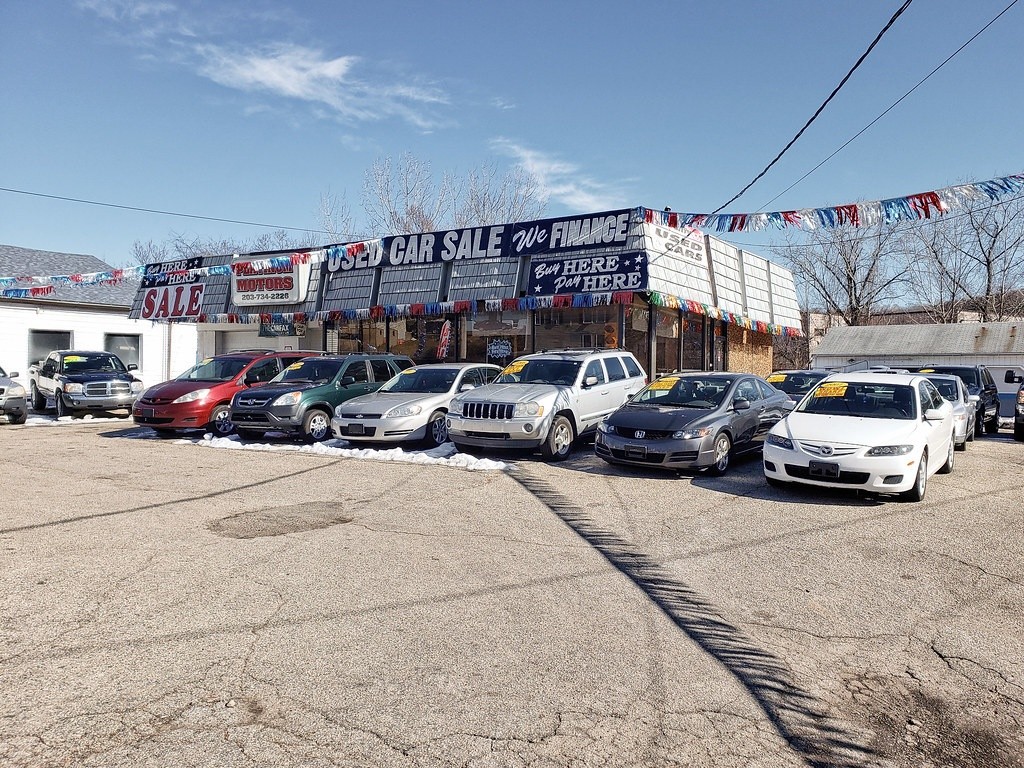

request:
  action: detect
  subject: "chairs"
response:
[671,377,911,418]
[65,362,113,373]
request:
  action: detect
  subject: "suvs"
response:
[131,347,328,437]
[445,348,649,462]
[229,352,419,442]
[915,363,1002,436]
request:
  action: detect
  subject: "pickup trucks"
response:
[25,350,144,419]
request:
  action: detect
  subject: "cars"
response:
[763,369,839,407]
[1005,370,1024,441]
[0,366,28,425]
[758,372,956,502]
[591,369,798,475]
[909,373,981,451]
[474,320,512,330]
[329,359,506,447]
